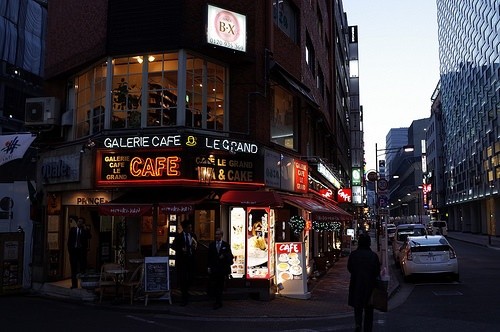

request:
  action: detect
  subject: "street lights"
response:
[374,142,415,177]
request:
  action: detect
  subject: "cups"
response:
[279,263,288,269]
[280,271,291,281]
[292,265,301,274]
[291,257,298,264]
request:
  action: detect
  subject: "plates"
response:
[248,239,268,267]
[278,252,302,283]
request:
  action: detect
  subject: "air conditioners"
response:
[25,96,61,126]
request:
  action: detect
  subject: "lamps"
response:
[274,284,284,298]
[85,137,104,152]
[80,145,96,154]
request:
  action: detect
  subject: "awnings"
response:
[98,190,211,217]
[220,190,283,207]
[282,194,352,220]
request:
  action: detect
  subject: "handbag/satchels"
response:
[372,275,389,312]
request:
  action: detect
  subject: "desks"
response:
[105,270,129,304]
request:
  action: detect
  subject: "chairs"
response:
[87,106,126,134]
[99,264,145,306]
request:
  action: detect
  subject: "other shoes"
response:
[213,303,222,310]
[70,285,77,289]
[181,300,189,307]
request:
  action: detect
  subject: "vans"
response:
[379,220,449,269]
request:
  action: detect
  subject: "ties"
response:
[217,241,221,253]
[185,233,190,255]
[77,228,80,246]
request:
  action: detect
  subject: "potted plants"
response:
[78,268,99,301]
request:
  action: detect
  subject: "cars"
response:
[398,235,458,284]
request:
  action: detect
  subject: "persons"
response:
[436,226,443,235]
[207,228,232,308]
[68,217,92,289]
[347,233,381,332]
[368,221,371,229]
[172,220,198,306]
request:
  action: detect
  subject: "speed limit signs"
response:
[378,178,388,191]
